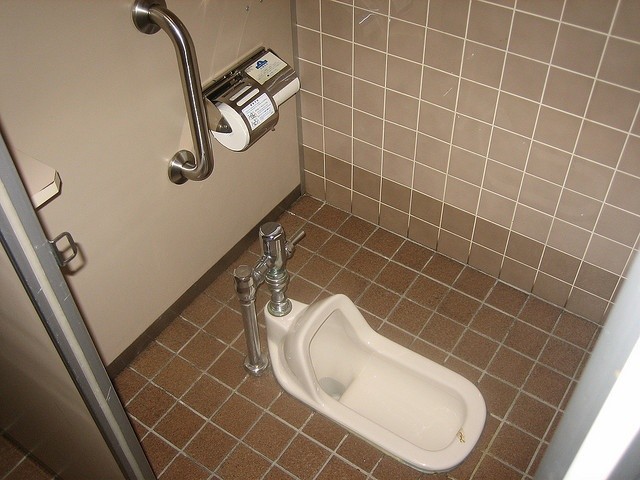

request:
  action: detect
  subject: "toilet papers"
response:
[196,48,300,152]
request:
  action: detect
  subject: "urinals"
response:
[263,292,487,474]
